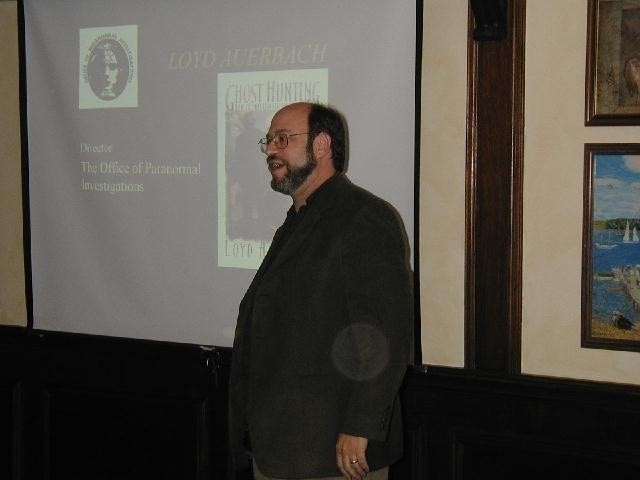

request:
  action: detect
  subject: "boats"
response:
[595,242,618,249]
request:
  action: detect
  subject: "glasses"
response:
[257,132,310,153]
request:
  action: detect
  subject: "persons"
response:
[226,102,421,480]
[89,40,124,98]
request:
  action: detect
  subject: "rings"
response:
[351,460,359,464]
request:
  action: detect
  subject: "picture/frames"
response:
[581,7,640,351]
